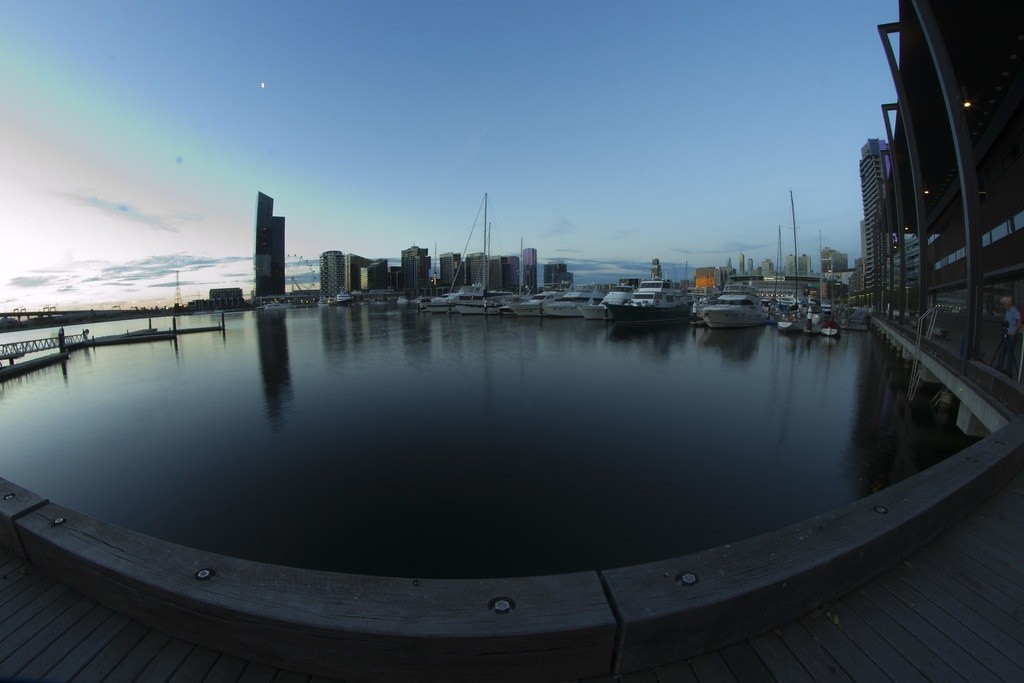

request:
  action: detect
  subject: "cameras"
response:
[1000,321,1009,328]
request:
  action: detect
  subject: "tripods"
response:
[990,326,1020,380]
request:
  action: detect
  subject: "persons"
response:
[997,295,1021,376]
[82,329,86,339]
[802,287,817,297]
[61,326,64,342]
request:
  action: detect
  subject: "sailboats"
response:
[454,191,505,315]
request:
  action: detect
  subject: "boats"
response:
[602,273,695,323]
[498,305,518,316]
[335,291,355,306]
[263,302,297,310]
[777,322,804,333]
[810,315,839,342]
[545,280,600,318]
[699,283,767,329]
[578,284,637,321]
[425,280,479,314]
[509,290,569,318]
